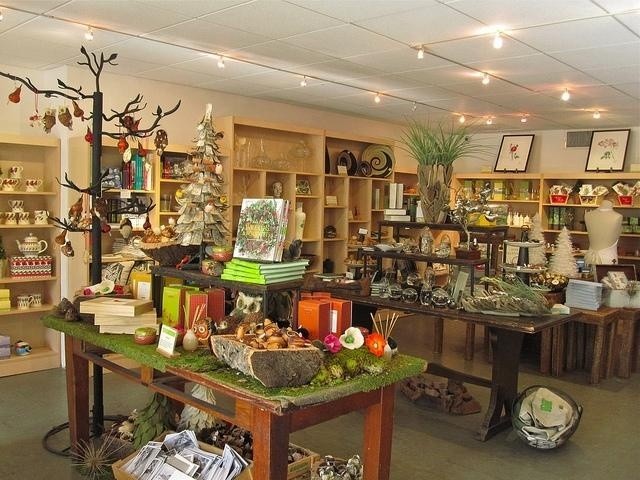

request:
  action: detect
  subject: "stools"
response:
[553,307,619,385]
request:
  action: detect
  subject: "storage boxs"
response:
[109,430,252,480]
[196,419,320,480]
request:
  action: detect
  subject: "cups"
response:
[161,193,172,212]
[30,294,42,307]
[17,296,33,310]
[8,199,24,212]
[18,212,31,225]
[26,179,42,193]
[4,212,17,225]
[2,179,18,191]
[10,166,24,179]
[34,210,49,225]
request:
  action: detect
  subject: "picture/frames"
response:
[585,129,630,172]
[494,134,535,172]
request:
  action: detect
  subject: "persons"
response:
[584,200,623,265]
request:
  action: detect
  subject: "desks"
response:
[383,219,509,281]
[330,288,584,443]
[361,248,490,296]
[42,314,427,480]
[149,264,305,332]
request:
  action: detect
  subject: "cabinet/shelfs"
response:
[68,136,231,375]
[543,173,640,259]
[394,168,420,207]
[450,173,542,240]
[0,135,62,377]
[214,116,324,275]
[323,131,395,275]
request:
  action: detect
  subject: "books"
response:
[222,196,312,284]
[80,293,162,341]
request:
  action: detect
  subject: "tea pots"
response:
[15,233,49,257]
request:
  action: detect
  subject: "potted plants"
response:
[396,112,498,225]
